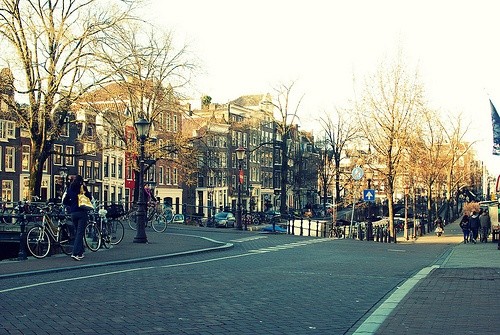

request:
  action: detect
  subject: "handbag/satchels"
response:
[77,185,95,211]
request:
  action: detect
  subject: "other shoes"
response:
[474,240,477,244]
[71,254,80,261]
[77,253,85,259]
[472,238,475,242]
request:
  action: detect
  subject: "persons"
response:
[68,175,93,261]
[435,216,444,233]
[460,208,491,244]
[143,184,157,221]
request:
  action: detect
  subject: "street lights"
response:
[235,144,247,230]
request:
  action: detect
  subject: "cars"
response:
[212,212,236,229]
[241,202,333,228]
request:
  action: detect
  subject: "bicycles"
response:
[0,196,174,259]
